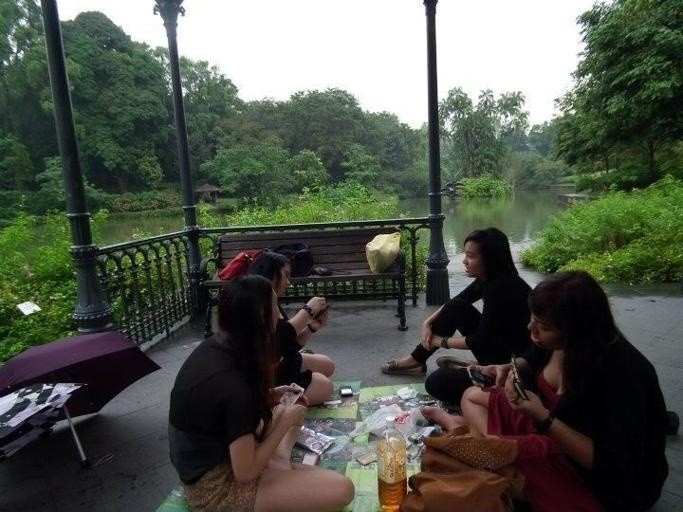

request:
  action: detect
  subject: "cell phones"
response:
[468,369,494,385]
[341,386,353,396]
[299,450,320,466]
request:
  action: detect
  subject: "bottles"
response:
[377,417,408,511]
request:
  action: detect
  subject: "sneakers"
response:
[436,355,474,370]
[302,349,318,357]
[381,358,426,378]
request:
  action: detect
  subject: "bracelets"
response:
[539,410,556,431]
[441,337,449,349]
[303,304,317,320]
[307,323,318,333]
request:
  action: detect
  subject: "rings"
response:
[480,365,484,369]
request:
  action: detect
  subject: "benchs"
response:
[199,227,408,338]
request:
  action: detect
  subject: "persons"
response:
[379,225,534,416]
[418,268,672,511]
[217,246,337,409]
[167,273,356,511]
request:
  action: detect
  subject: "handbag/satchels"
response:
[226,242,315,281]
[410,433,528,512]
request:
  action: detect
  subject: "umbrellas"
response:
[0,329,161,465]
